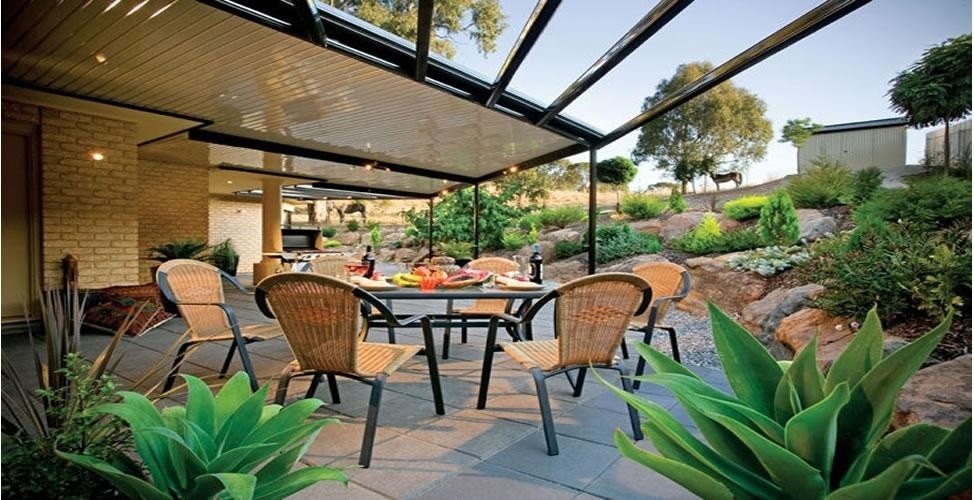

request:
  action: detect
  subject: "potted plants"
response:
[213,234,240,276]
[145,238,213,317]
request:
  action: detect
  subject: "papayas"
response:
[441,273,479,289]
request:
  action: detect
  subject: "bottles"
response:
[360,245,376,278]
[527,244,543,285]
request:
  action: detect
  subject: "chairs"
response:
[441,257,524,360]
[301,255,395,346]
[254,272,446,469]
[156,259,285,400]
[476,271,654,456]
[620,262,691,390]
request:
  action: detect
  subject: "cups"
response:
[513,255,527,280]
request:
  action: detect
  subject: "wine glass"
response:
[398,249,413,271]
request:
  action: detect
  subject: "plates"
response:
[503,283,544,291]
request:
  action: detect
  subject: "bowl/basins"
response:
[344,264,370,277]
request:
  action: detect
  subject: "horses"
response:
[329,201,366,224]
[709,170,742,191]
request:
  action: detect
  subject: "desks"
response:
[278,274,558,382]
